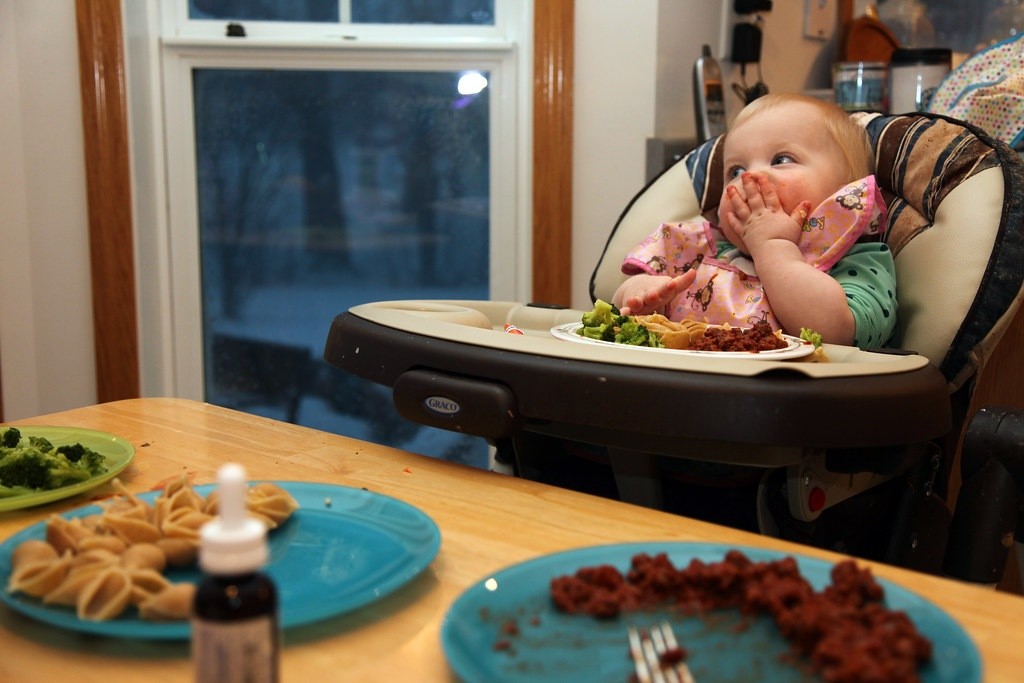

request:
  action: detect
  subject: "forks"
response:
[625,619,699,683]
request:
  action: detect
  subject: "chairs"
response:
[321,112,1024,575]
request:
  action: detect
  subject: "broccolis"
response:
[798,327,822,349]
[0,427,109,491]
[575,299,665,348]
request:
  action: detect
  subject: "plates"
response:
[0,480,442,643]
[0,425,136,512]
[549,319,816,360]
[440,540,985,683]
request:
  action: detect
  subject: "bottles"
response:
[188,463,281,683]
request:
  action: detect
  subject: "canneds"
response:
[830,61,888,114]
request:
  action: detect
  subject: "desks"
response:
[0,396,1024,683]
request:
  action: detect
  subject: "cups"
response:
[834,63,885,114]
[890,44,953,117]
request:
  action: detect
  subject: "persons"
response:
[608,93,898,511]
